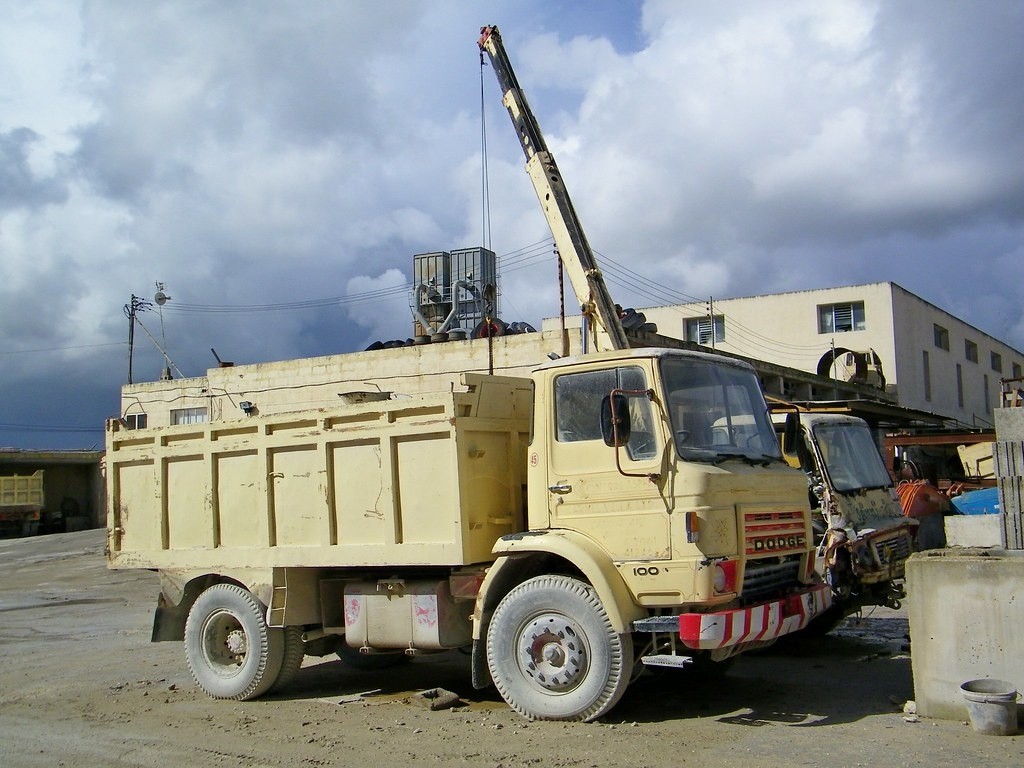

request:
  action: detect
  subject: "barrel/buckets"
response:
[960,678,1023,736]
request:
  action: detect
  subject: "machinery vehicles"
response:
[881,428,997,551]
[677,406,922,641]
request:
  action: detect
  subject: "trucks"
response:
[101,346,842,723]
[0,469,45,537]
